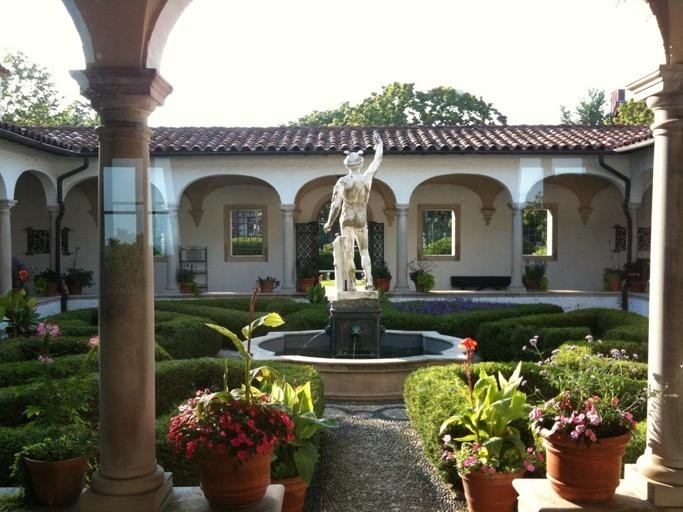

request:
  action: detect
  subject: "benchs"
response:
[451,276,512,291]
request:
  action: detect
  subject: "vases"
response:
[22,454,90,505]
[457,469,525,512]
[538,422,632,503]
[199,453,270,509]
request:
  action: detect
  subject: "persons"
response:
[324,133,383,291]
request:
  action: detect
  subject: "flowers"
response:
[521,335,683,449]
[168,388,296,470]
[9,319,99,477]
[440,337,544,480]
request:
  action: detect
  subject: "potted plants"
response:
[602,269,624,290]
[11,255,28,299]
[65,246,96,295]
[298,263,317,292]
[176,269,196,293]
[33,268,66,296]
[522,257,549,291]
[407,252,435,292]
[256,276,280,293]
[241,366,339,512]
[372,265,392,292]
